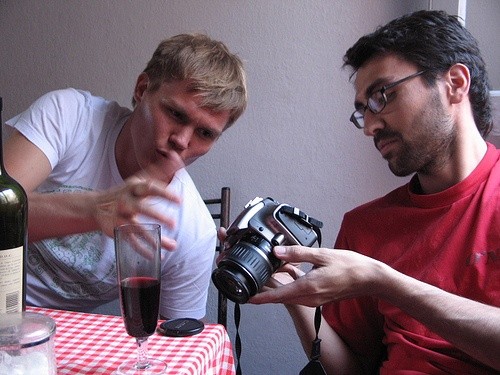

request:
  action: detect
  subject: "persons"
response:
[217,10,500,375]
[0,34,247,324]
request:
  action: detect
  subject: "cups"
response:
[0,311,56,375]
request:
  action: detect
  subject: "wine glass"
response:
[114,224,167,375]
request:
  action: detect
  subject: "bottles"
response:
[0,97,29,327]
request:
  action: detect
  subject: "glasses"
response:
[349,70,426,129]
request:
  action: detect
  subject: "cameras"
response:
[212,196,318,304]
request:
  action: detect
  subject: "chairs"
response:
[201,186,232,333]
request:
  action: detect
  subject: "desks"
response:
[0,301,236,375]
